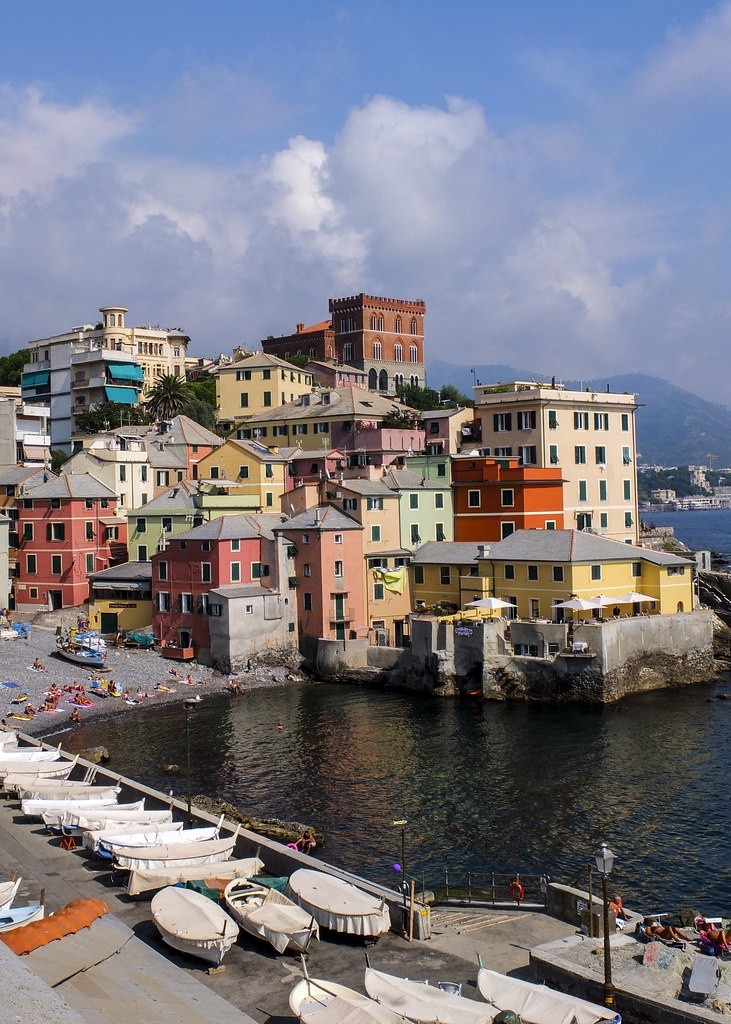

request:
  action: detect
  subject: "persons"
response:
[6,703,39,719]
[187,674,199,688]
[89,669,155,703]
[643,917,693,943]
[154,683,176,692]
[300,831,317,854]
[612,604,649,618]
[695,917,731,952]
[15,692,27,700]
[77,611,91,633]
[34,657,49,672]
[116,625,124,642]
[231,679,244,693]
[199,676,208,684]
[169,668,183,677]
[71,707,85,722]
[0,607,14,628]
[1,719,23,731]
[165,638,173,647]
[38,682,94,711]
[609,896,631,923]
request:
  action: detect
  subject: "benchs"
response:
[692,917,731,956]
[637,921,687,951]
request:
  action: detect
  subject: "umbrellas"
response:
[587,594,623,618]
[549,597,608,624]
[616,591,661,617]
[464,596,519,616]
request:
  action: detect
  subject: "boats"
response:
[129,846,266,902]
[61,800,176,835]
[287,952,416,1024]
[363,952,522,1024]
[16,777,124,802]
[3,765,99,795]
[56,635,107,669]
[288,867,391,940]
[0,729,63,764]
[475,952,622,1024]
[0,871,23,912]
[150,885,240,966]
[99,813,226,861]
[21,798,118,822]
[224,872,320,954]
[111,822,242,880]
[42,796,148,834]
[82,821,184,857]
[0,887,46,934]
[0,752,81,787]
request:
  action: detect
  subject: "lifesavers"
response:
[509,881,523,902]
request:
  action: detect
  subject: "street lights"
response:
[593,841,618,1012]
